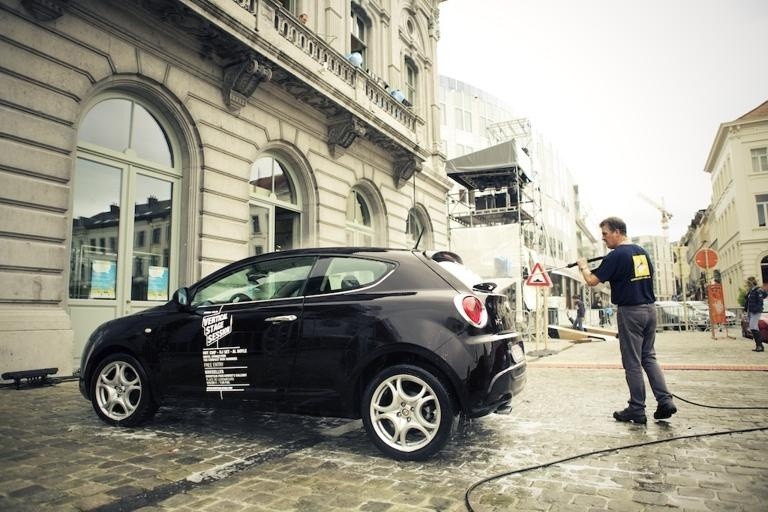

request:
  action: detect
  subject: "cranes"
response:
[638,192,673,229]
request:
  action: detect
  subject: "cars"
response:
[655,301,736,331]
[741,298,768,343]
[79,227,526,461]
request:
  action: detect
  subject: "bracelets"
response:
[580,266,588,271]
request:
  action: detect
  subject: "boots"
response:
[751,329,764,352]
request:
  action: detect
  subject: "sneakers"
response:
[653,400,677,419]
[613,407,647,424]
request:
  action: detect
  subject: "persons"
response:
[575,215,678,426]
[591,296,602,308]
[570,294,586,330]
[299,13,308,24]
[742,276,765,352]
